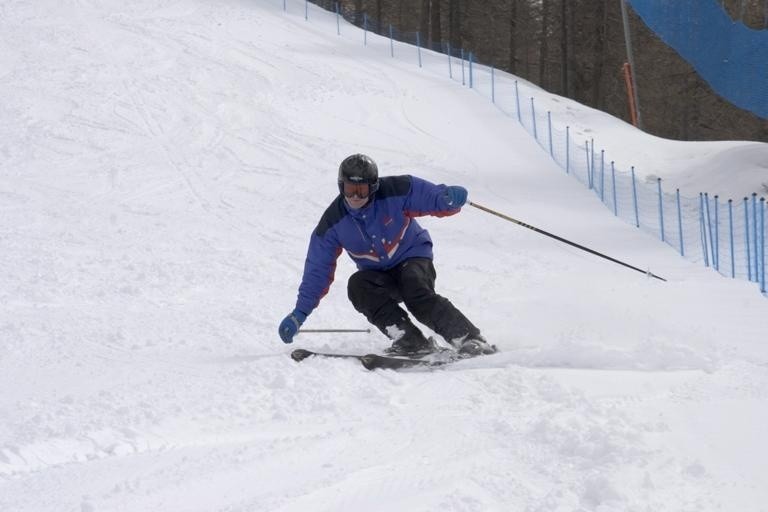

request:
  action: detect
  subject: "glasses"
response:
[338,179,379,199]
[338,155,378,193]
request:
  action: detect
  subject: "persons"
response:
[279,153,496,356]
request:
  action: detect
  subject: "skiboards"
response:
[291,350,500,371]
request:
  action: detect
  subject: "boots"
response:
[385,317,438,356]
[452,333,493,355]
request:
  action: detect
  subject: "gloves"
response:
[445,187,466,208]
[278,309,307,343]
